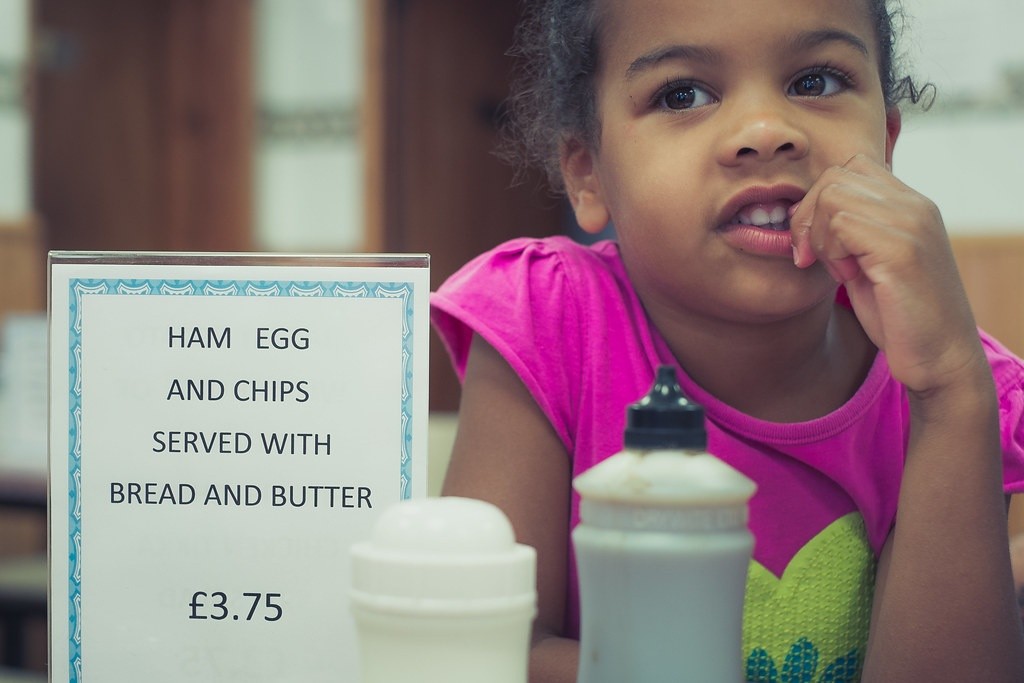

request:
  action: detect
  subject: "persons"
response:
[426,0,1023,681]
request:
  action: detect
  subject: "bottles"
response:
[338,362,760,682]
